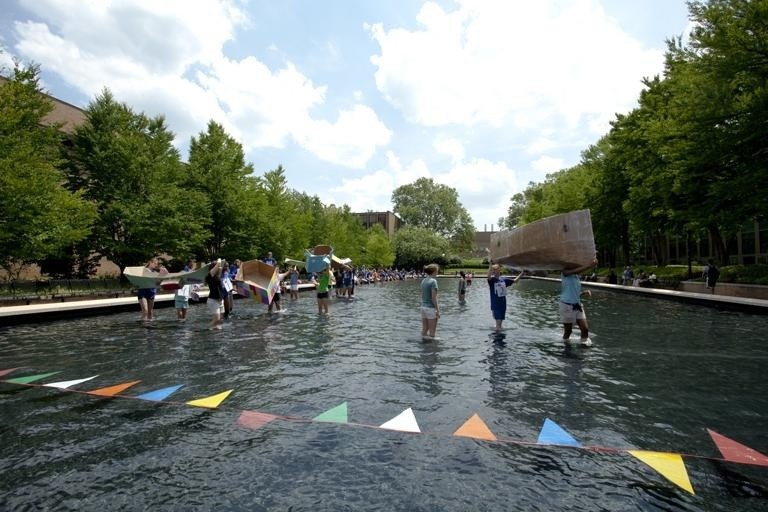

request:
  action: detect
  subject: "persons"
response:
[607,262,657,287]
[454,268,475,302]
[486,255,524,330]
[558,260,591,345]
[133,251,416,329]
[420,263,442,339]
[702,258,721,295]
